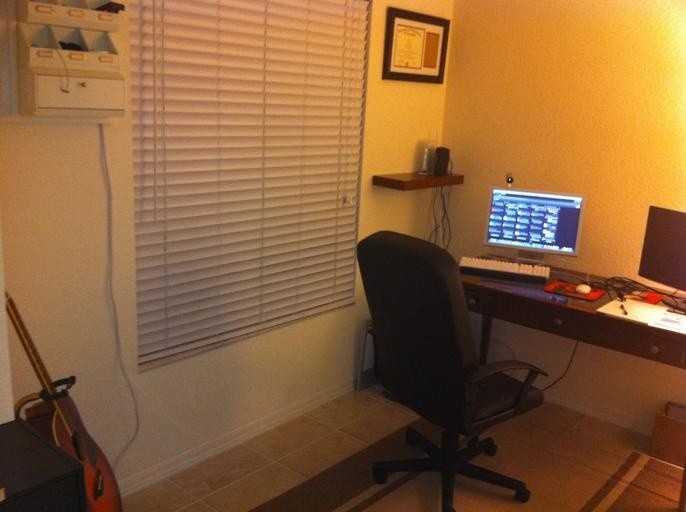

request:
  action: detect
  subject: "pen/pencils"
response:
[621,304,627,315]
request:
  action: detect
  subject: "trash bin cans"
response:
[649,401,686,468]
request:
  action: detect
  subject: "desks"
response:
[459,250,685,450]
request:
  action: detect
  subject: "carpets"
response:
[244,393,686,511]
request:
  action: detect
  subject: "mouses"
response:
[576,283,591,295]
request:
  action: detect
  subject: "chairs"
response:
[354,229,551,512]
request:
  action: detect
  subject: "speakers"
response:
[0,418,88,511]
[434,147,450,177]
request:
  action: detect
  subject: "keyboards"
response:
[458,255,551,283]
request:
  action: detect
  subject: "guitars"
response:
[4,288,125,512]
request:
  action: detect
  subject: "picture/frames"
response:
[381,5,451,83]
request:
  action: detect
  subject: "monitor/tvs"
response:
[481,186,586,264]
[637,205,686,312]
